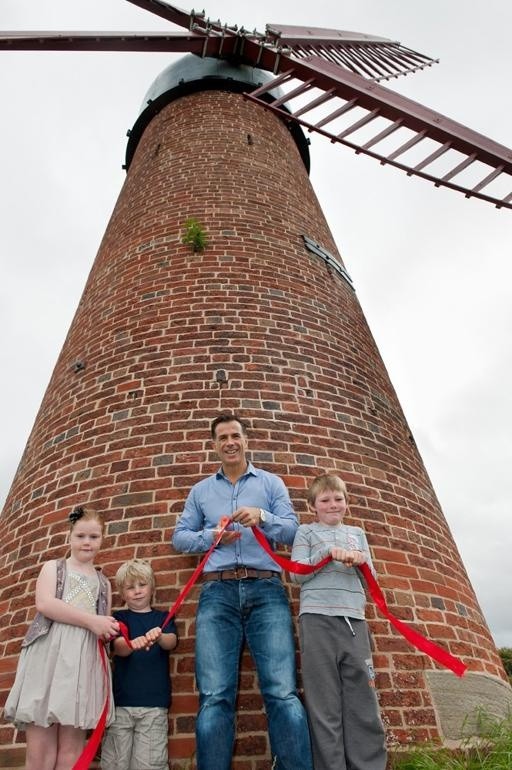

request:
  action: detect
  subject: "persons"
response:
[4,507,121,770]
[172,414,314,770]
[99,558,180,770]
[290,473,388,770]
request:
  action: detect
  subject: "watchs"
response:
[259,508,266,525]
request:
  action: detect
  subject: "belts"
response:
[199,569,283,582]
